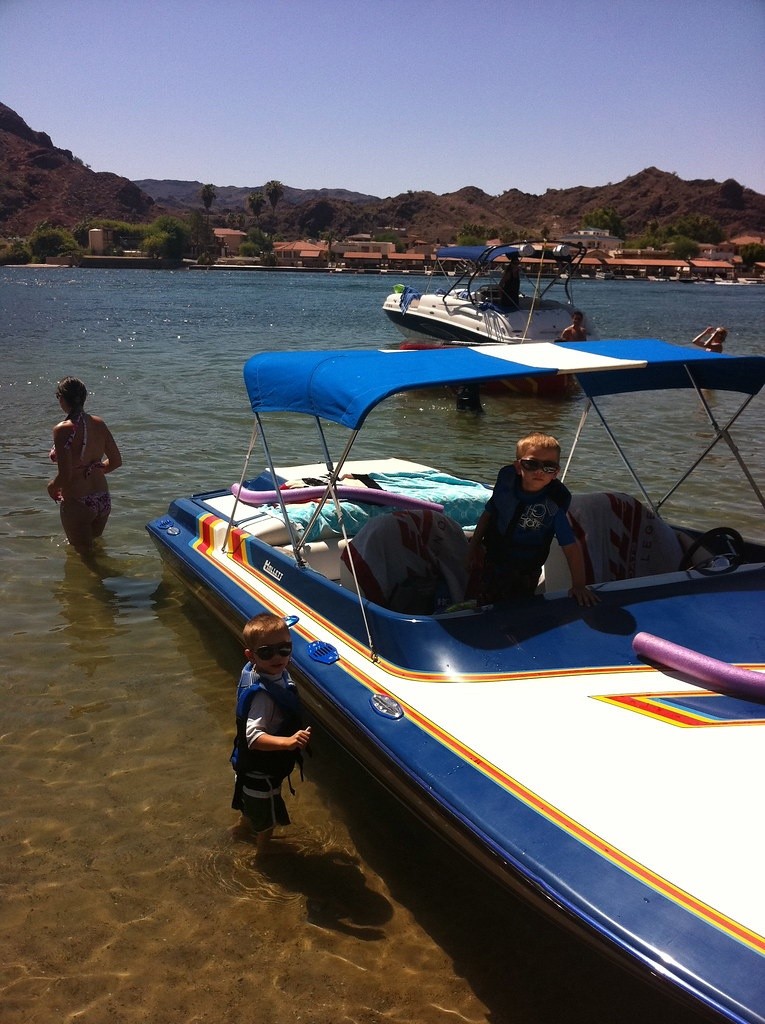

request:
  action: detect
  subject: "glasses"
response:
[517,458,559,474]
[254,641,292,660]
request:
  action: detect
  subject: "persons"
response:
[470,431,603,609]
[497,257,520,313]
[229,614,313,836]
[560,310,589,341]
[692,326,727,353]
[47,376,121,547]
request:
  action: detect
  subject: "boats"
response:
[148,327,765,1024]
[400,338,578,399]
[553,274,568,285]
[716,273,758,285]
[382,239,602,341]
[677,279,700,283]
[595,269,615,281]
